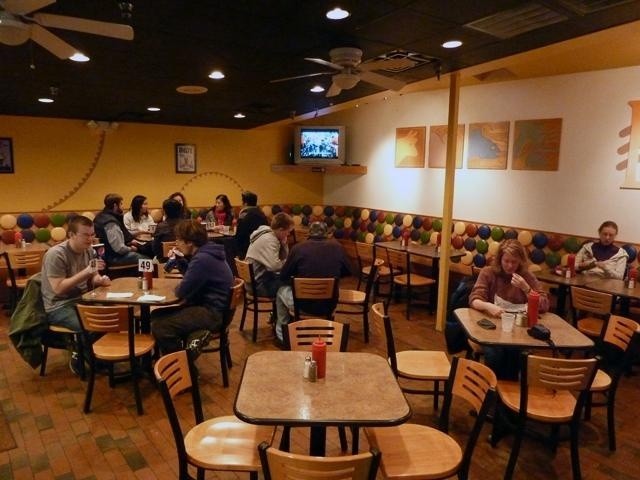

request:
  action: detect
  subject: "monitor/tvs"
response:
[293,125,346,164]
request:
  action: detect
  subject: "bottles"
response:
[516,310,528,328]
[312,335,327,378]
[15,230,27,249]
[304,355,311,378]
[395,225,442,253]
[308,361,317,382]
[624,264,637,288]
[526,287,540,328]
[555,253,577,280]
[137,271,154,291]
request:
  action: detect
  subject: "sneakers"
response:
[76,232,96,238]
[68,338,85,376]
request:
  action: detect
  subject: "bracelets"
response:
[527,287,532,294]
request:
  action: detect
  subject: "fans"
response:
[269,45,414,98]
[1,0,135,60]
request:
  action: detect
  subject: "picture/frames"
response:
[0,137,14,174]
[175,142,197,174]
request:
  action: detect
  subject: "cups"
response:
[198,212,238,236]
[501,313,515,332]
[91,243,105,270]
[163,249,185,274]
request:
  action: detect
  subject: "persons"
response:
[557,219,629,359]
[198,191,356,351]
[38,214,113,382]
[466,236,551,423]
[93,192,192,278]
[143,219,237,397]
